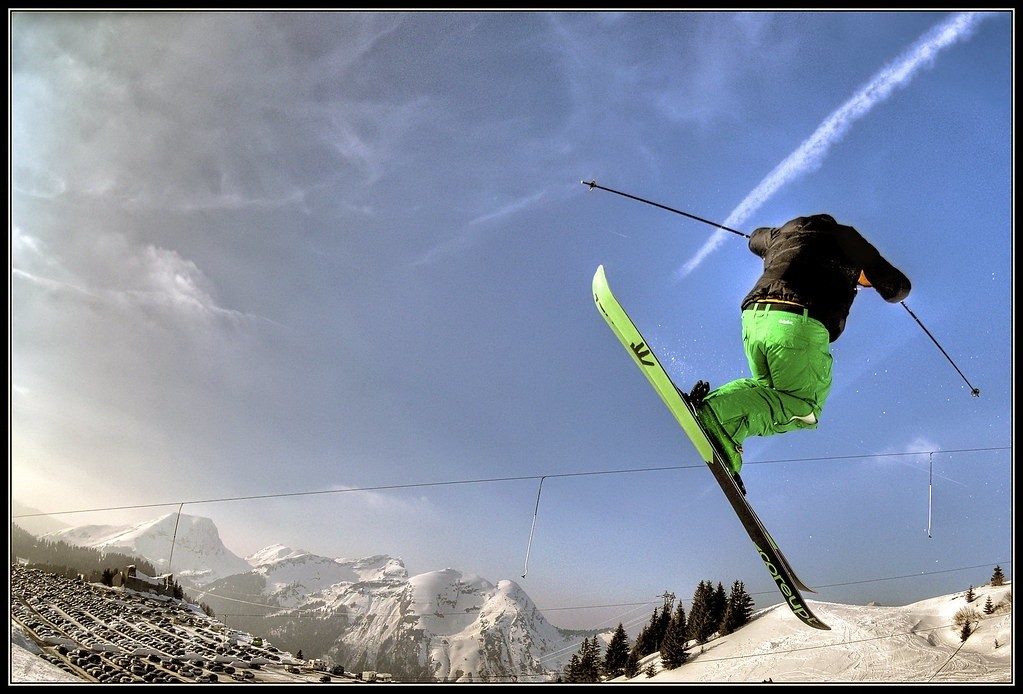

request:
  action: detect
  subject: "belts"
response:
[745,302,818,320]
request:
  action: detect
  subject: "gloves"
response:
[858,269,873,287]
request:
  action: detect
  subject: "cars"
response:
[195,675,210,683]
[267,648,278,652]
[205,661,224,672]
[39,646,204,684]
[231,673,244,681]
[249,663,261,669]
[189,668,203,676]
[264,654,273,659]
[224,667,235,674]
[269,656,280,660]
[264,647,272,650]
[242,655,253,661]
[241,670,255,679]
[12,564,267,657]
[289,668,300,674]
[207,673,218,682]
[319,676,331,682]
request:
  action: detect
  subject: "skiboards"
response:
[591,264,833,631]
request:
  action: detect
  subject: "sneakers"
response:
[699,402,742,473]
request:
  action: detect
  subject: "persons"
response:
[689,212,913,473]
